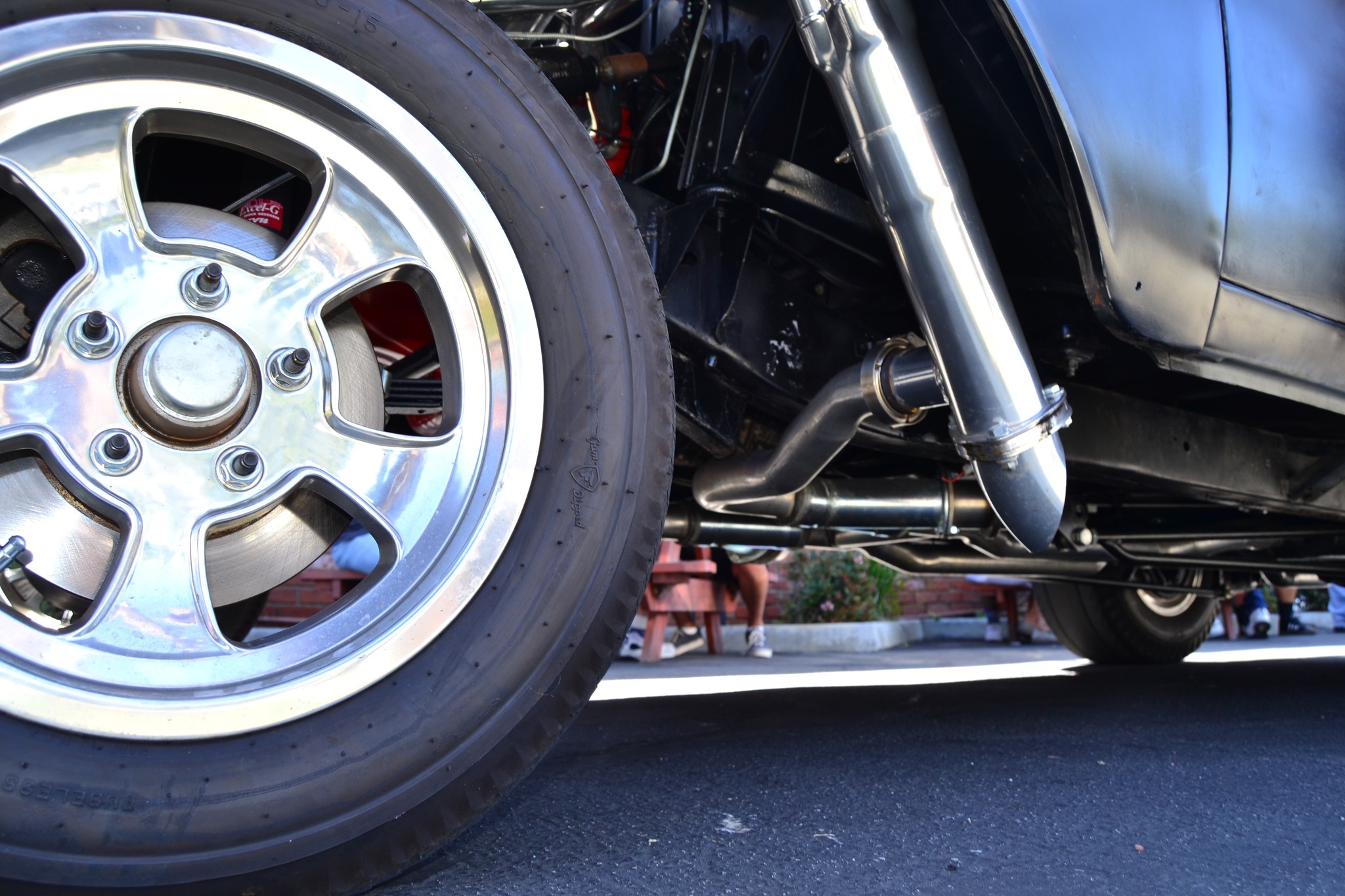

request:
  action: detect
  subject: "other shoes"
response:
[1019,617,1057,642]
[1208,616,1225,638]
[983,621,1004,642]
[1245,607,1272,638]
[1334,625,1345,632]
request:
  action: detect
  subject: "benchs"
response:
[302,558,717,599]
[963,579,1035,606]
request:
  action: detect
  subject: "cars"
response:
[0,0,1343,896]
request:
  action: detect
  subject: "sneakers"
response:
[743,628,774,659]
[621,631,678,661]
[1280,616,1316,635]
[669,624,706,657]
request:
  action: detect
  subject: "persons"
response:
[1019,598,1062,643]
[1277,587,1317,635]
[982,589,1004,641]
[661,545,774,659]
[1211,587,1271,639]
[1327,583,1345,633]
